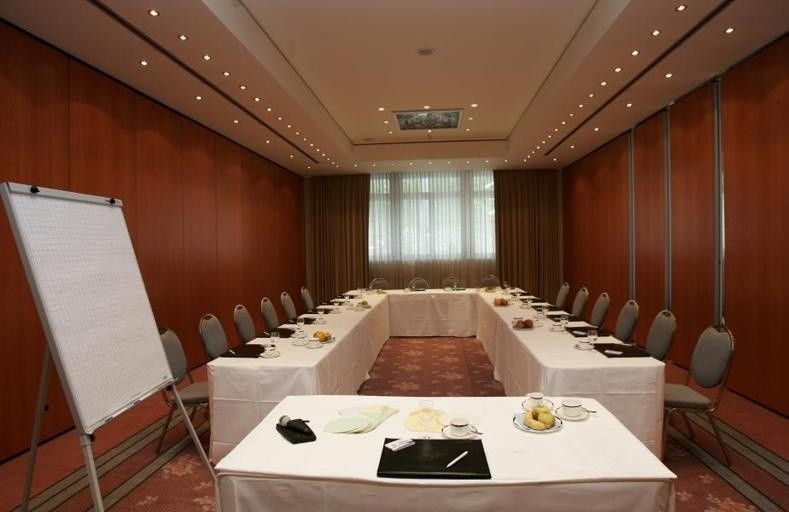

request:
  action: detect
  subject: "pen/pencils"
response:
[264,332,270,335]
[229,350,235,354]
[446,451,469,468]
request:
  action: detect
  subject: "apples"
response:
[314,331,331,342]
[515,319,532,329]
[532,405,554,428]
[494,298,508,306]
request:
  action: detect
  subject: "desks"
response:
[214,394,679,512]
[206,286,666,465]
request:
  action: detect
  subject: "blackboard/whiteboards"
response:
[0,182,174,434]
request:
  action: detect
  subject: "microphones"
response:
[278,415,311,435]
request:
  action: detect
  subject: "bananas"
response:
[523,408,545,432]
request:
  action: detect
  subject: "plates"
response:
[521,399,555,413]
[440,424,478,440]
[555,407,589,422]
[514,414,564,434]
[260,351,279,359]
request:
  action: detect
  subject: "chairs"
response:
[369,273,500,290]
[154,275,230,457]
[555,281,736,472]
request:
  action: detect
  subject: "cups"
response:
[526,391,545,409]
[561,400,583,416]
[450,416,468,437]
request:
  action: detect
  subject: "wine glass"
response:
[267,286,365,354]
[415,400,435,440]
[495,279,598,356]
